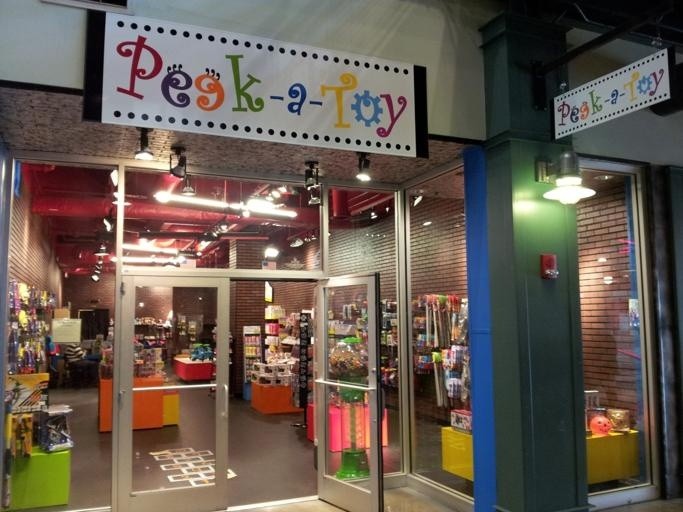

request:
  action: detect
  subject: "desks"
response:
[440,425,641,494]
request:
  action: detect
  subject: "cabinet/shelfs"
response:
[240,292,474,453]
[5,282,236,453]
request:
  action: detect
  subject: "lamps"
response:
[182,178,196,194]
[82,183,308,282]
[135,128,154,162]
[532,150,599,208]
[308,169,322,208]
[110,168,121,185]
[303,162,319,190]
[355,151,373,182]
[169,149,189,178]
